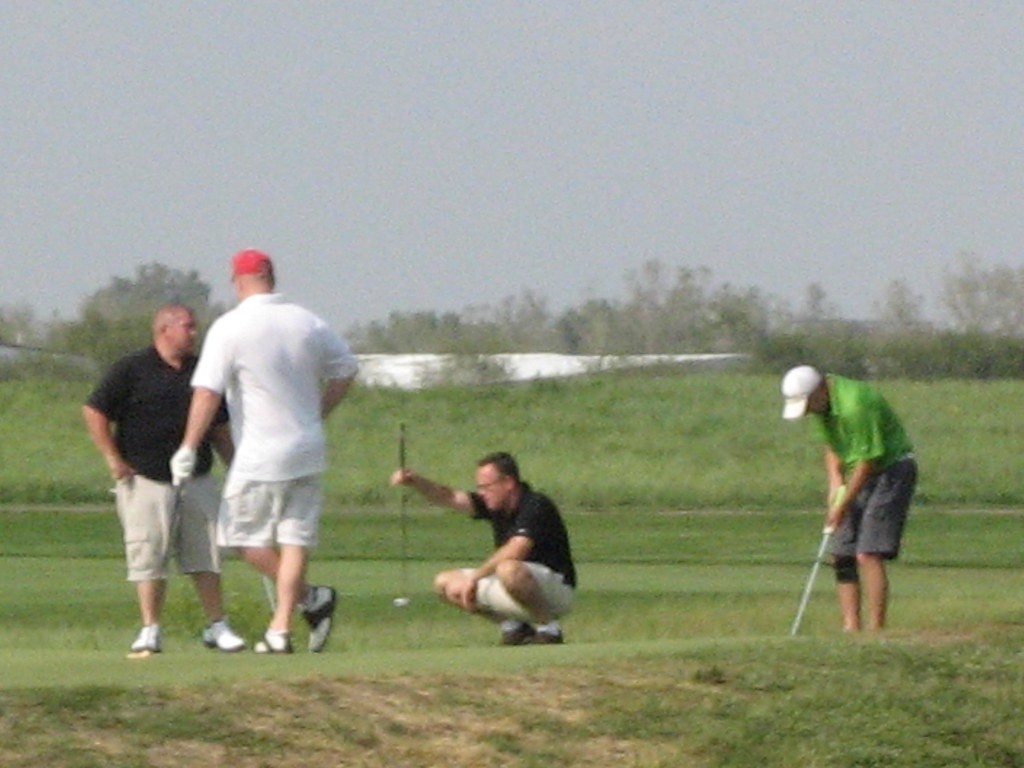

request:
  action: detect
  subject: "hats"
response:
[230,250,270,282]
[781,364,822,419]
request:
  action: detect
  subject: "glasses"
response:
[478,477,505,491]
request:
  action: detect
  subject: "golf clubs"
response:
[790,484,848,636]
[392,421,412,609]
[261,575,277,612]
[125,480,186,660]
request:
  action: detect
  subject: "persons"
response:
[781,364,920,634]
[390,453,577,643]
[82,301,246,653]
[169,250,362,652]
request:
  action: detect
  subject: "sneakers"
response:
[500,622,535,647]
[202,618,244,650]
[253,628,291,653]
[128,623,163,657]
[528,622,563,646]
[300,584,336,651]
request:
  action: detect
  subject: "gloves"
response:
[169,445,198,487]
